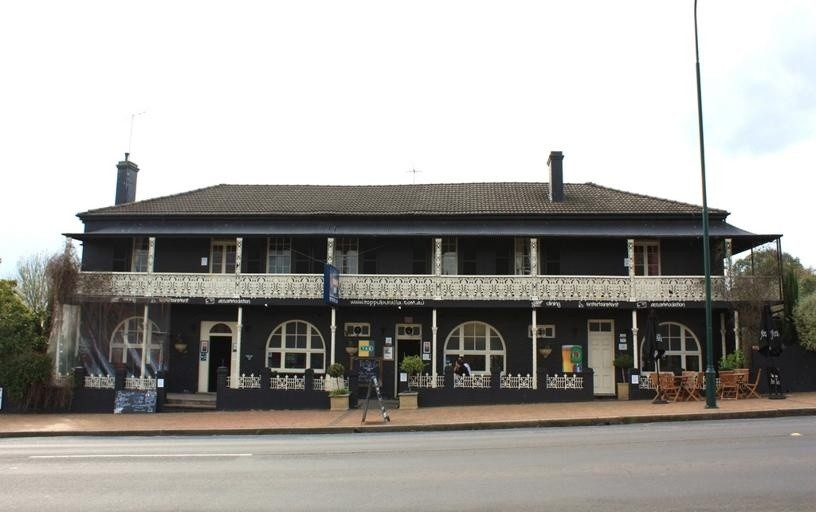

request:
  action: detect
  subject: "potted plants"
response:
[326,362,351,411]
[613,353,634,401]
[398,353,423,410]
[720,347,749,385]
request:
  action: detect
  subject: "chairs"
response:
[650,367,761,402]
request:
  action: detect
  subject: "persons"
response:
[454,359,469,377]
[458,356,471,376]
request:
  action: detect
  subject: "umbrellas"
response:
[643,303,664,387]
[757,303,786,375]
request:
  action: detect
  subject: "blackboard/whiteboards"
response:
[372,378,389,419]
[358,357,384,388]
[113,389,157,414]
